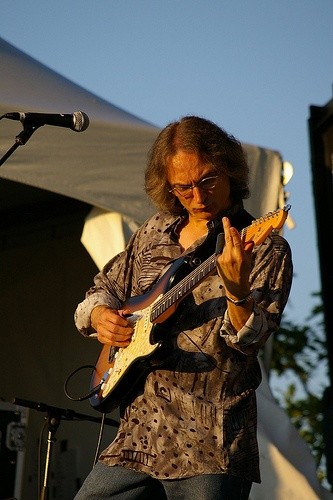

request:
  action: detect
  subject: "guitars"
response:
[85,202,292,414]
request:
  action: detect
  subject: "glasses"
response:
[167,169,221,196]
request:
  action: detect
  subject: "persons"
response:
[73,114,296,500]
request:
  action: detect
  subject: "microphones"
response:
[4,111,89,132]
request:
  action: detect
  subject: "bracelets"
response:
[223,287,256,307]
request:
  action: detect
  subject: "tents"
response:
[0,37,329,500]
